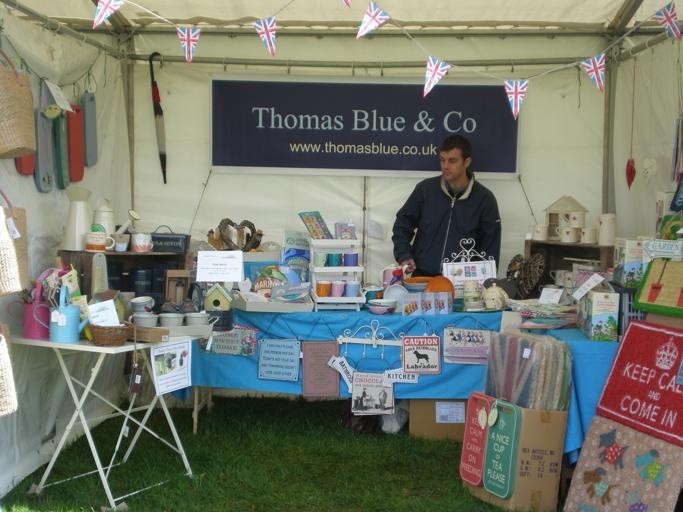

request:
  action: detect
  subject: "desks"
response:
[12,335,198,511]
[129,308,620,497]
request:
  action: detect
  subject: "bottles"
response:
[483,282,509,310]
[253,265,288,300]
[160,298,197,313]
[597,213,616,247]
[57,188,92,252]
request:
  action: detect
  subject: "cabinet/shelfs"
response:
[306,237,366,313]
[522,238,615,299]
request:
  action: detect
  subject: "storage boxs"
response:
[404,394,474,443]
[460,389,569,511]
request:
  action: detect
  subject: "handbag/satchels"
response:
[1,52,37,160]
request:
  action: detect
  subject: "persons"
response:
[391,134,500,280]
[359,387,367,411]
[377,388,387,410]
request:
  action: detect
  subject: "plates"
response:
[384,284,408,313]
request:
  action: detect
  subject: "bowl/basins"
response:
[368,299,397,315]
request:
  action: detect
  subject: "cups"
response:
[326,252,359,267]
[407,290,452,315]
[84,232,115,253]
[110,234,129,252]
[364,288,382,303]
[206,310,232,331]
[554,209,597,244]
[157,313,184,328]
[549,269,573,288]
[126,296,158,328]
[463,280,484,308]
[131,233,151,253]
[538,284,558,293]
[316,278,359,298]
[185,311,209,327]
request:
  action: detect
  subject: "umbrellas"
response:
[148,50,170,186]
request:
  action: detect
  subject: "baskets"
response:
[89,324,133,347]
